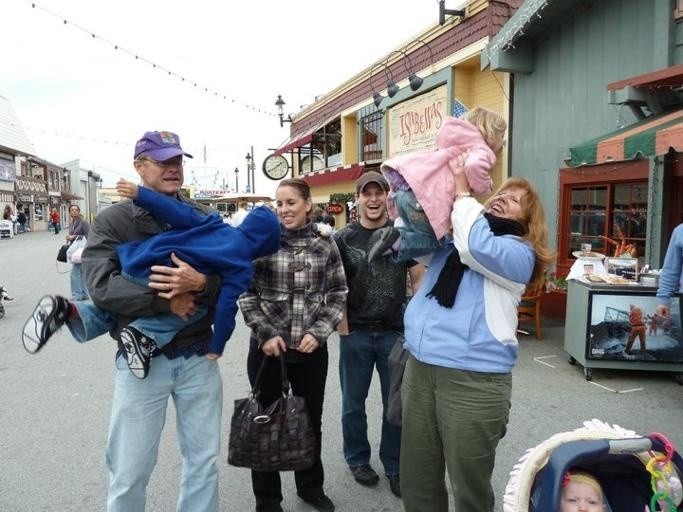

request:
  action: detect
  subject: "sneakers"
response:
[310,494,335,512]
[120,326,157,379]
[22,295,69,354]
[387,475,401,498]
[368,226,400,264]
[350,464,379,487]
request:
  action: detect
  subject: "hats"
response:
[355,172,390,193]
[134,131,193,162]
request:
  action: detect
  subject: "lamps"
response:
[385,51,412,98]
[370,63,393,108]
[404,39,433,91]
[275,95,292,127]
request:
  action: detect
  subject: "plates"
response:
[572,251,605,261]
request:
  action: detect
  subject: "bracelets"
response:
[453,191,471,202]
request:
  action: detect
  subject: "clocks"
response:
[263,154,289,180]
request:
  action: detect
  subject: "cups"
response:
[581,243,592,256]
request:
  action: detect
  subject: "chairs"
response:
[517,281,544,340]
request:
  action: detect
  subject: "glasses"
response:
[137,159,186,169]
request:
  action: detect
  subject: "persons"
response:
[400,176,556,512]
[332,171,425,498]
[231,201,250,229]
[21,177,284,380]
[624,303,673,355]
[50,206,60,234]
[655,223,683,386]
[81,130,222,512]
[561,471,609,512]
[3,206,26,233]
[314,207,336,225]
[239,178,350,512]
[368,106,507,265]
[66,205,90,301]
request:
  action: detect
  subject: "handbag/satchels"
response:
[228,397,316,470]
[57,245,69,262]
